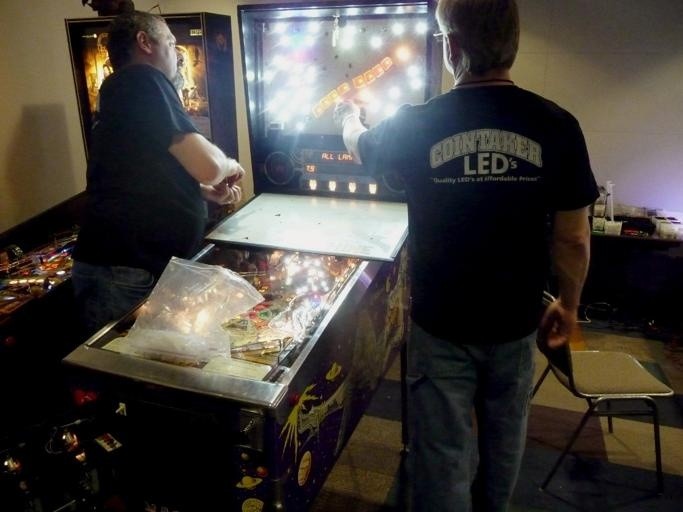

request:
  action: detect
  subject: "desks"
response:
[577,213,683,322]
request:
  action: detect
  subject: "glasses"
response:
[432,30,451,41]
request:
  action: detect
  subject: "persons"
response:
[71,11,245,347]
[335,0,601,511]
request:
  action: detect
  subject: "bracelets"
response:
[342,113,359,127]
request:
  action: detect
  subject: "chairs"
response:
[529,290,675,492]
